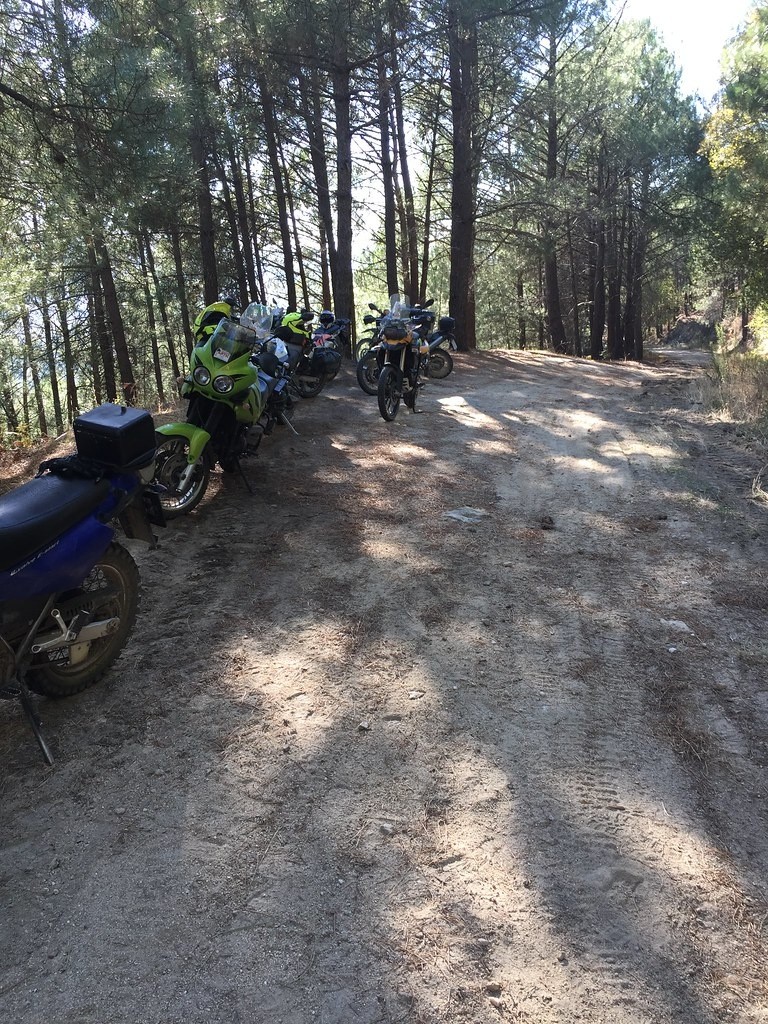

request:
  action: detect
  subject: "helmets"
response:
[319,310,334,329]
[282,312,309,338]
[194,302,231,341]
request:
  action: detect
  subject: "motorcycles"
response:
[0,403,168,768]
[150,296,351,520]
[355,293,458,422]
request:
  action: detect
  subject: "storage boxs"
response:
[71,403,157,472]
